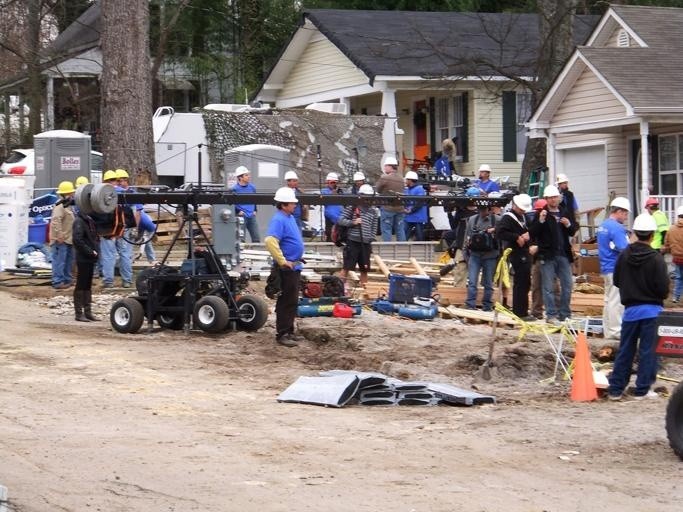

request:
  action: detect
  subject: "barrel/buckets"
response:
[332,302,353,318]
[28,219,49,244]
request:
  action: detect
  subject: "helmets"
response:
[479,164,492,172]
[513,174,569,212]
[56,167,129,194]
[357,183,374,195]
[353,172,365,181]
[631,212,657,232]
[326,172,339,181]
[405,171,419,180]
[284,171,298,180]
[644,198,659,208]
[235,166,250,177]
[610,197,631,212]
[274,187,299,203]
[677,205,683,215]
[384,156,398,165]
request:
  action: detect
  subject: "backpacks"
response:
[331,204,357,246]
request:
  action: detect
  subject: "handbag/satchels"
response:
[466,229,498,252]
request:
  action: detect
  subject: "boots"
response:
[74,290,91,322]
[83,290,102,321]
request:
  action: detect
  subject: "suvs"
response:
[0,147,103,186]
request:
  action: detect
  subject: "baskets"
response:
[388,274,432,304]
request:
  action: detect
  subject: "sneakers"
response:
[122,282,130,288]
[627,387,648,396]
[57,279,77,289]
[606,391,622,401]
[276,332,305,347]
[520,315,538,322]
[99,283,113,288]
[546,318,560,325]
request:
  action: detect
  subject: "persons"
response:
[337,184,378,303]
[441,138,458,176]
[605,213,671,403]
[230,165,262,243]
[441,164,580,326]
[71,176,89,279]
[97,170,144,277]
[49,180,76,289]
[645,197,670,308]
[597,196,639,341]
[100,170,138,289]
[664,206,683,303]
[134,201,157,263]
[261,186,306,348]
[70,210,103,323]
[320,156,427,246]
[284,171,309,237]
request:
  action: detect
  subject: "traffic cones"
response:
[566,332,600,404]
